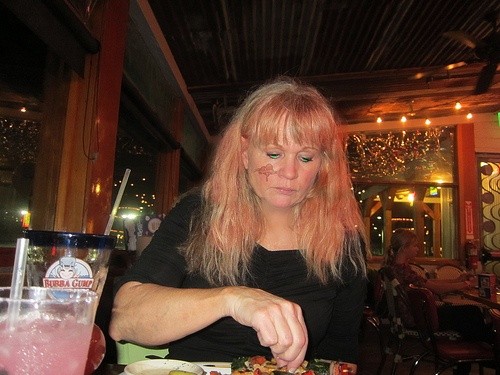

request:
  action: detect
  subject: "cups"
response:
[0,286,98,375]
[24,229,117,307]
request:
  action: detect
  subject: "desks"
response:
[464,289,500,311]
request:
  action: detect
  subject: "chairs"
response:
[363,260,500,375]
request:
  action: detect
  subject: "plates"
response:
[124,359,203,375]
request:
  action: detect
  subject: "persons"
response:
[108,77,371,375]
[383,229,484,338]
